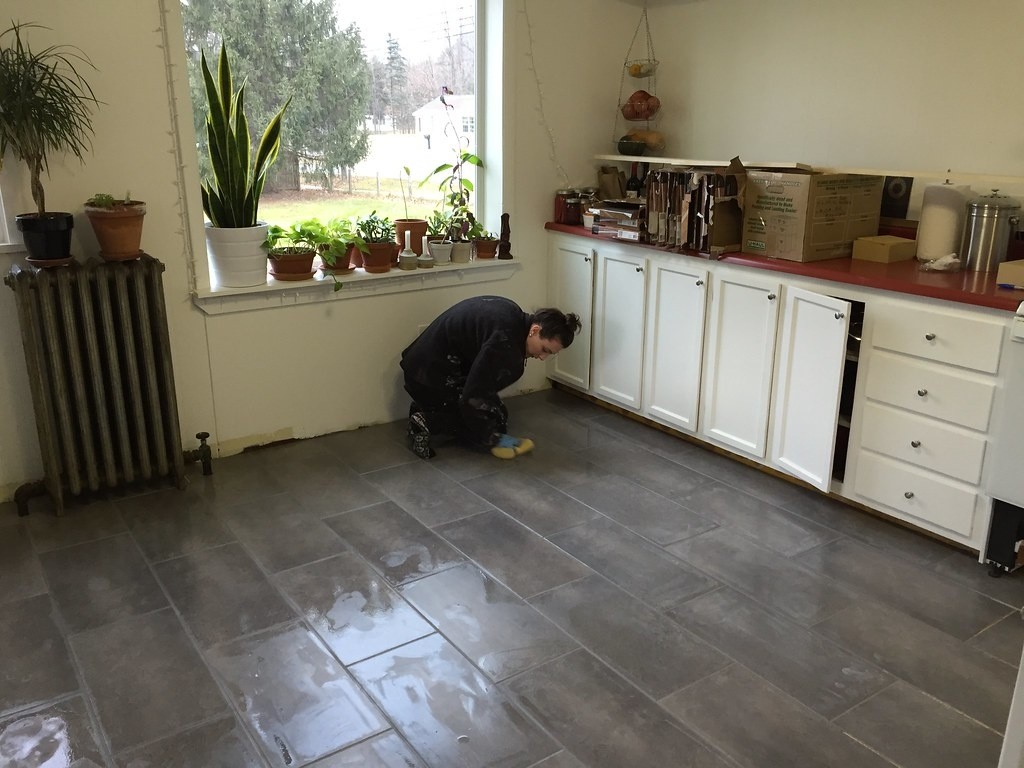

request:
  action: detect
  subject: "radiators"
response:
[3,254,214,517]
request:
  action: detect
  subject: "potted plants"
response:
[356,208,397,273]
[258,218,344,292]
[419,86,484,265]
[306,216,372,276]
[472,229,501,259]
[84,192,148,262]
[425,207,450,241]
[391,164,428,257]
[198,41,293,291]
[427,217,462,266]
[0,19,110,258]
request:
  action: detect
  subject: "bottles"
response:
[583,187,599,205]
[574,189,585,198]
[580,198,590,223]
[566,198,580,225]
[625,162,642,199]
[554,189,574,223]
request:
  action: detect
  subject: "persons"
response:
[399,294,583,459]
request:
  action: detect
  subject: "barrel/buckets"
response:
[913,180,971,262]
[959,188,1023,273]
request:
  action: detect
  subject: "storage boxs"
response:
[741,168,886,264]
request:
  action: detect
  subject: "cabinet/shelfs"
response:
[546,220,600,399]
[839,280,1014,561]
[611,6,662,154]
[770,264,872,504]
[700,256,785,468]
[648,246,717,440]
[594,237,651,421]
